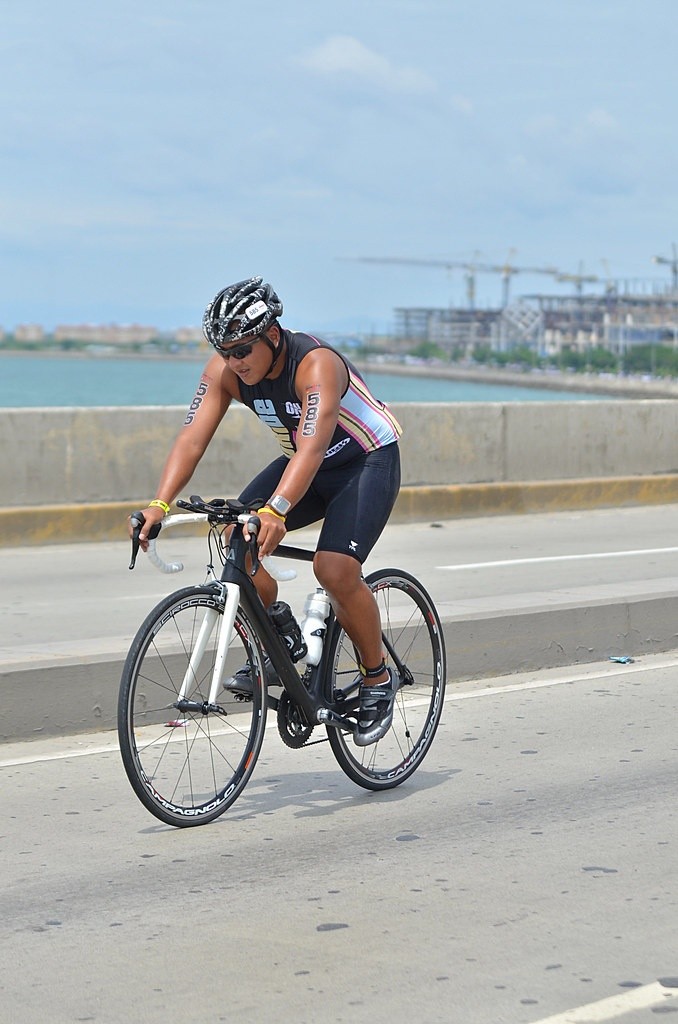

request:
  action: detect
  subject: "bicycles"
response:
[116,497,447,827]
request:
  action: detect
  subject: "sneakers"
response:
[353,667,399,747]
[222,649,282,692]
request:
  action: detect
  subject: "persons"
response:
[127,275,403,746]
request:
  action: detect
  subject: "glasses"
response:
[213,321,277,360]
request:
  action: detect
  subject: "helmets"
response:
[203,276,283,345]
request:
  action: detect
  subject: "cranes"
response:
[355,258,601,319]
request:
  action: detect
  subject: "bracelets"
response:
[258,508,285,522]
[149,500,170,517]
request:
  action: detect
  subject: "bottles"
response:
[266,600,308,661]
[300,587,331,667]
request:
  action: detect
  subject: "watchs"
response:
[265,495,292,517]
